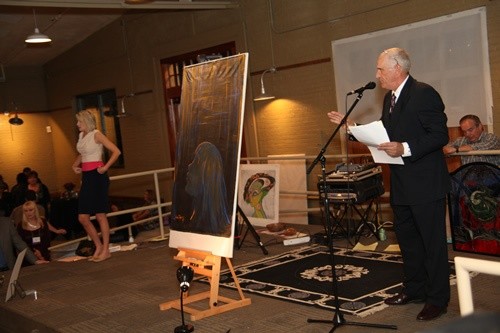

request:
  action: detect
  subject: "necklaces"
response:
[29,223,40,228]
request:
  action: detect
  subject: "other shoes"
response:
[88,256,95,261]
[93,253,111,262]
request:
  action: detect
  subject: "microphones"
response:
[347,82,376,96]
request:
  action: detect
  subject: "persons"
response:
[327,48,450,321]
[72,109,121,261]
[0,166,159,231]
[443,114,500,241]
[15,203,66,261]
[0,213,50,272]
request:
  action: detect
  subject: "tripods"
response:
[305,92,397,333]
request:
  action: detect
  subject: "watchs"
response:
[453,145,460,152]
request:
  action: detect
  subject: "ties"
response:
[390,93,396,113]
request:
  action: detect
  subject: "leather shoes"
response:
[417,303,447,321]
[384,292,424,305]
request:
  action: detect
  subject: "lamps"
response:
[174,265,196,333]
[25,7,52,43]
[254,66,277,101]
[117,92,136,117]
[8,101,24,126]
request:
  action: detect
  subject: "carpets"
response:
[194,243,480,319]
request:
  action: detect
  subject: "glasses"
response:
[382,48,398,65]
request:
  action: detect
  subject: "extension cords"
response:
[284,236,310,245]
